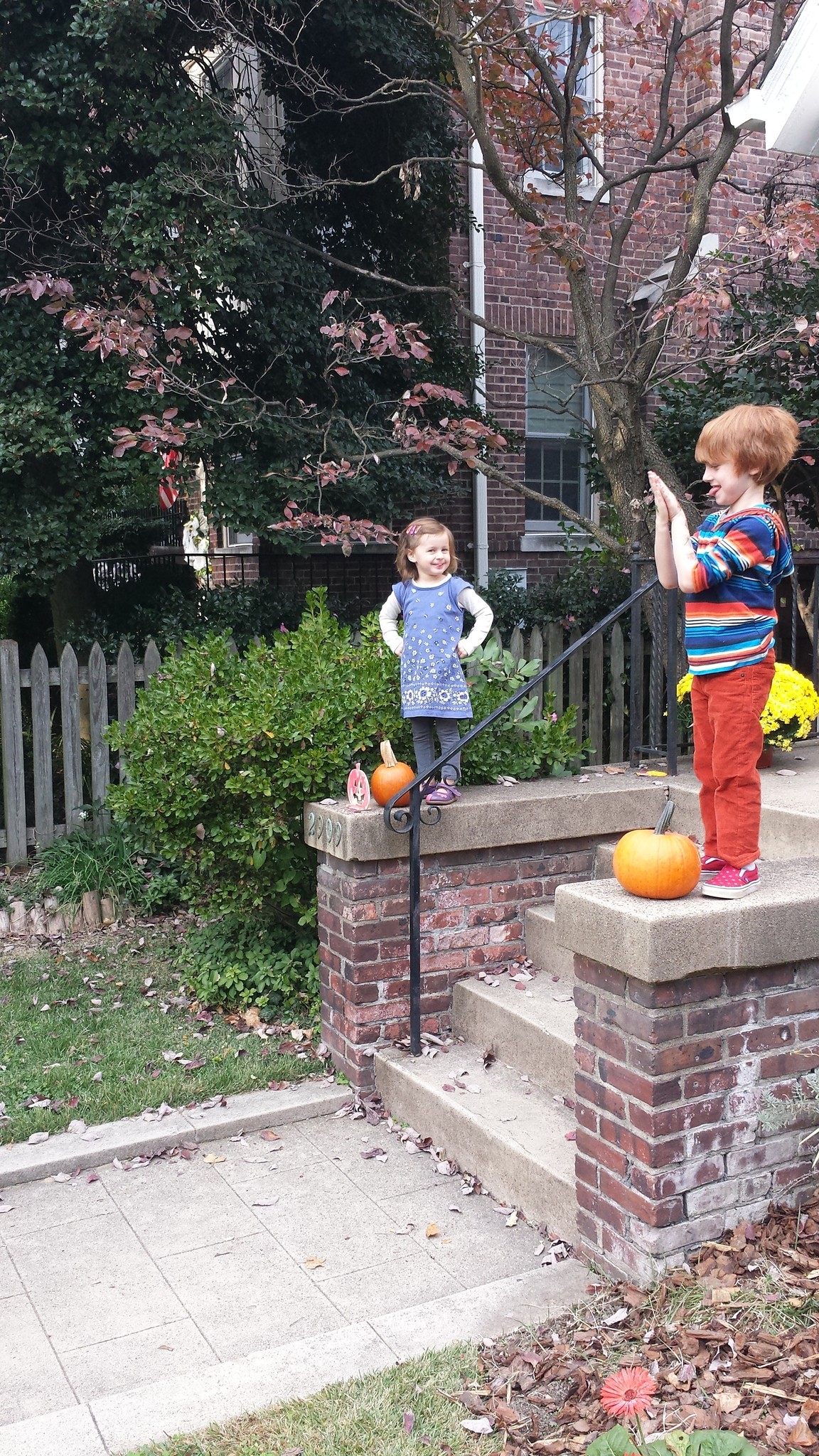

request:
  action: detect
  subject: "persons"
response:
[646,402,802,904]
[378,516,497,805]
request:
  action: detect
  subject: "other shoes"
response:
[426,783,462,805]
[702,864,761,899]
[419,780,439,799]
[697,855,729,882]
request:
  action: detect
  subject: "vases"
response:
[753,743,776,771]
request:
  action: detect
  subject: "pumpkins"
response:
[611,799,702,899]
[371,739,418,806]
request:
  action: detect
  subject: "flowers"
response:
[661,659,819,756]
